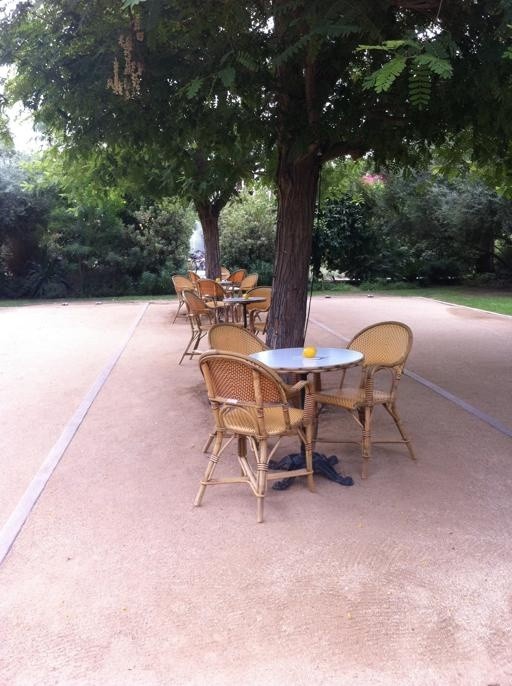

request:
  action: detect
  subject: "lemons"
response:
[303,346,316,359]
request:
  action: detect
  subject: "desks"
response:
[247,347,365,490]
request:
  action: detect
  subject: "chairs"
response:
[314,320,417,479]
[202,324,299,452]
[172,264,272,365]
[193,349,318,523]
